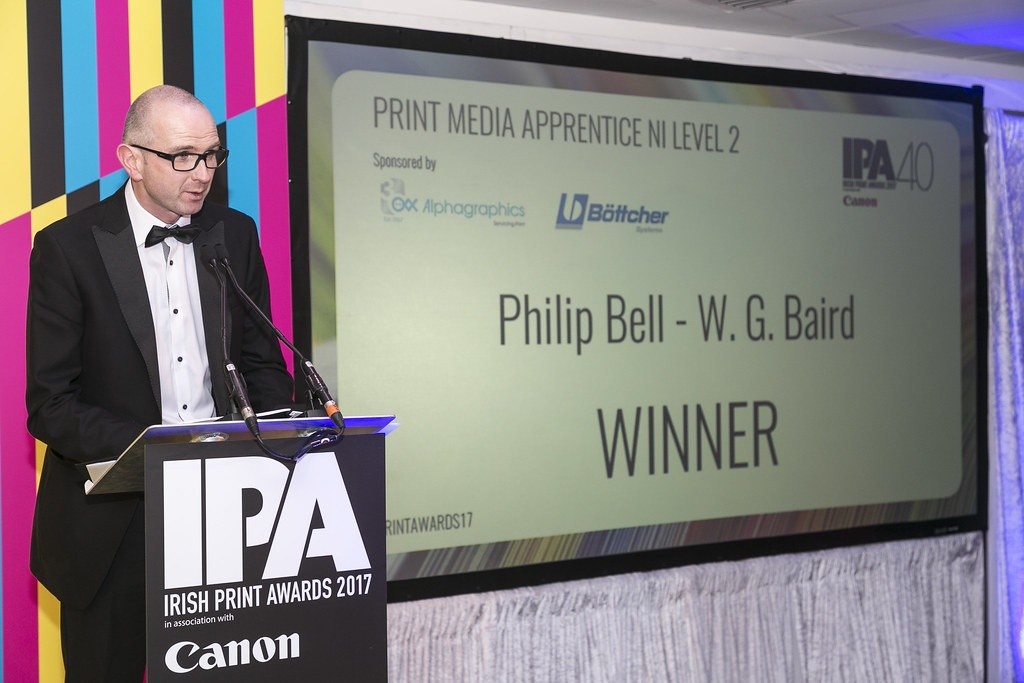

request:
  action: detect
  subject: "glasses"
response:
[130,144,229,172]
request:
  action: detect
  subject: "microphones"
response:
[199,242,345,436]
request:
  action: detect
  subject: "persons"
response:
[25,85,295,683]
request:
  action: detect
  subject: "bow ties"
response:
[145,223,201,248]
